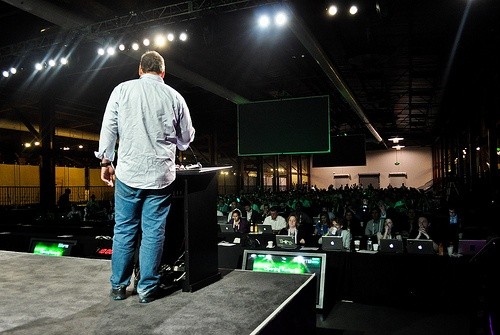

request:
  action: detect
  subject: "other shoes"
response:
[139,287,160,302]
[112,288,127,300]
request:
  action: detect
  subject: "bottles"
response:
[250,222,253,232]
[367,237,373,251]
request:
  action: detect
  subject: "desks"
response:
[243,244,465,315]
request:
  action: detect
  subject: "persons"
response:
[262,207,288,230]
[242,205,260,226]
[377,216,404,246]
[316,214,331,234]
[277,213,309,248]
[339,211,362,231]
[448,206,462,223]
[227,201,242,222]
[217,182,445,217]
[66,206,84,220]
[58,189,71,208]
[86,194,100,210]
[93,50,194,302]
[408,216,439,253]
[288,203,313,225]
[365,208,385,234]
[318,218,353,249]
[317,206,336,219]
[227,210,250,233]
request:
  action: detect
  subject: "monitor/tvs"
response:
[243,249,327,309]
[29,237,77,256]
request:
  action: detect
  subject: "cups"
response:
[447,246,453,255]
[255,226,257,232]
[372,244,379,251]
[438,243,444,256]
[268,241,274,248]
[354,241,361,251]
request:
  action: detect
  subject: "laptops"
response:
[257,224,272,233]
[322,236,344,249]
[276,235,298,249]
[406,239,435,254]
[380,238,404,253]
[458,240,486,254]
[220,224,234,232]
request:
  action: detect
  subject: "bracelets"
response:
[99,161,112,167]
